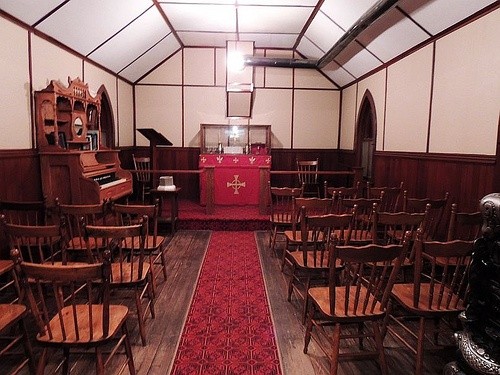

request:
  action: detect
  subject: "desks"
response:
[149,187,182,234]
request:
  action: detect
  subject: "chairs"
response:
[270,157,480,375]
[132,154,150,201]
[0,196,167,375]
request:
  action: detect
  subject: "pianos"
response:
[39,150,133,237]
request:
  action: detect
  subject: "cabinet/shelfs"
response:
[34,76,102,155]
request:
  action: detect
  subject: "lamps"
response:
[229,0,245,72]
